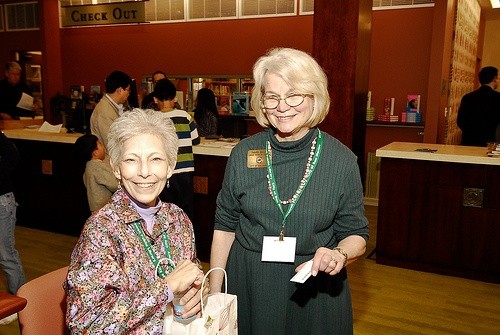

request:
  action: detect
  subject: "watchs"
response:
[333,246,347,261]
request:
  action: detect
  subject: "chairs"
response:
[15,266,70,335]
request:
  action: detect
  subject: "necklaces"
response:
[265,137,318,205]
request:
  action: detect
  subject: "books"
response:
[366,108,420,122]
[486,143,500,156]
[406,94,421,113]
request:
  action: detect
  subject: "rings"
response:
[331,259,337,264]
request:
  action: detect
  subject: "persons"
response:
[63,108,209,335]
[456,66,500,148]
[71,70,217,218]
[1,61,43,297]
[409,99,417,109]
[209,45,371,335]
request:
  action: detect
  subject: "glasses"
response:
[124,88,131,93]
[260,93,313,110]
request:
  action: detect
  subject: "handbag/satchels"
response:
[154,257,239,335]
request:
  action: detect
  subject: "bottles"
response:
[407,100,416,124]
[174,288,196,324]
